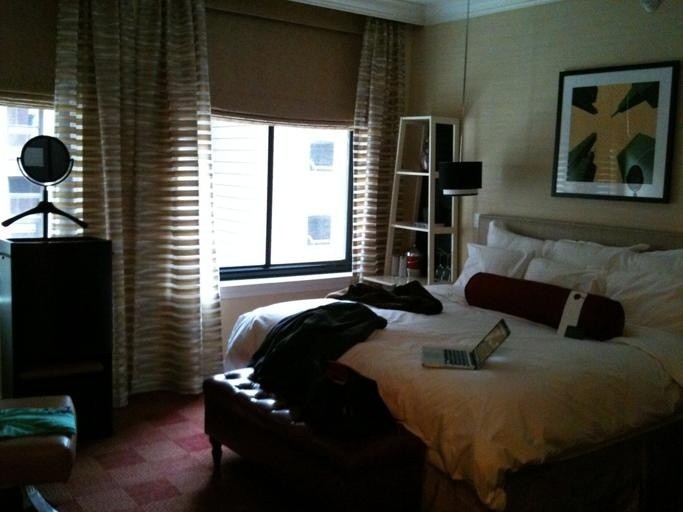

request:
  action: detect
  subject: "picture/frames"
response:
[551,61,678,203]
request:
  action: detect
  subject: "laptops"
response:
[422,318,510,368]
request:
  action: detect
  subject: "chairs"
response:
[0,394,77,511]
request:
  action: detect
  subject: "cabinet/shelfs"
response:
[0,236,111,443]
[363,115,457,292]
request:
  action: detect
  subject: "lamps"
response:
[438,1,482,197]
[641,0,664,13]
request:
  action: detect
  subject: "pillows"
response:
[436,221,683,342]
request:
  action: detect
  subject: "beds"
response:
[228,213,682,512]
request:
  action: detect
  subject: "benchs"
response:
[202,366,427,512]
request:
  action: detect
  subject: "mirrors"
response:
[2,134,88,241]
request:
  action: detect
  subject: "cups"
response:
[391,250,422,278]
